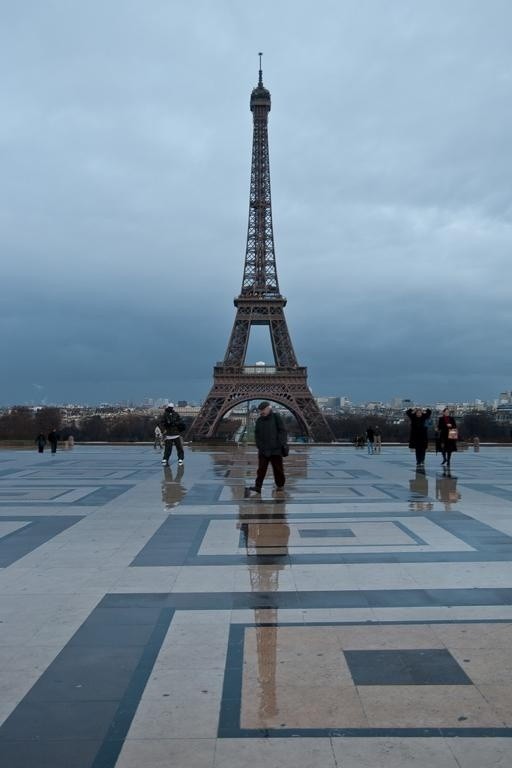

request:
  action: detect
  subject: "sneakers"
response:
[178,459,183,462]
[161,459,168,463]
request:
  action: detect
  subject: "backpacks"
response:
[448,429,458,439]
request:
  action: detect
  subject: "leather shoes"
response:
[249,486,261,493]
[441,460,450,465]
[277,484,284,491]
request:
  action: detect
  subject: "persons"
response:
[249,401,288,494]
[154,424,164,449]
[47,428,59,453]
[36,430,46,453]
[406,408,432,465]
[435,409,457,465]
[366,426,375,454]
[162,403,184,466]
[373,423,384,450]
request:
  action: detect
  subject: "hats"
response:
[259,402,270,410]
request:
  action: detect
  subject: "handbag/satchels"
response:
[281,443,289,457]
